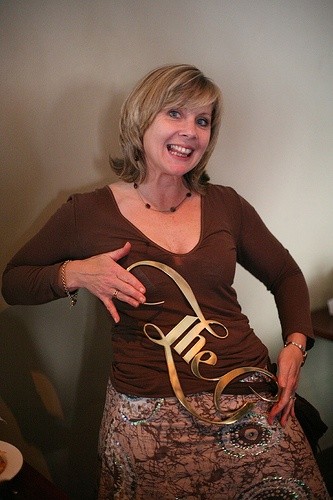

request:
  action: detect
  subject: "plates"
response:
[0,440,24,482]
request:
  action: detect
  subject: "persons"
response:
[0,61,332,500]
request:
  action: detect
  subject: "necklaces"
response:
[132,178,193,213]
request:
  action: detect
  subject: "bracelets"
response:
[283,340,307,367]
[61,260,78,306]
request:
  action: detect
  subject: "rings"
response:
[288,395,296,402]
[112,289,119,300]
